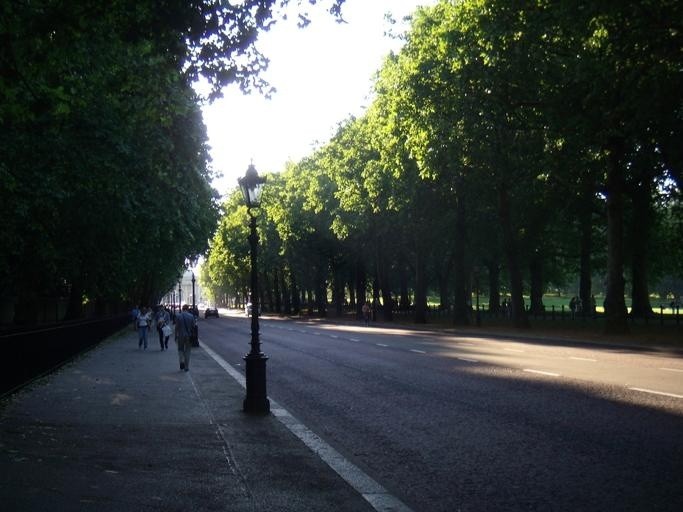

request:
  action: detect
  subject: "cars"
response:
[182,304,199,316]
[204,307,219,318]
[245,302,262,317]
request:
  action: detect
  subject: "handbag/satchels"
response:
[162,325,173,337]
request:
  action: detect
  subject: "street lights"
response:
[173,255,198,311]
[239,161,269,415]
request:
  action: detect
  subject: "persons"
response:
[362,302,370,323]
[569,297,576,321]
[175,304,195,372]
[132,305,170,351]
